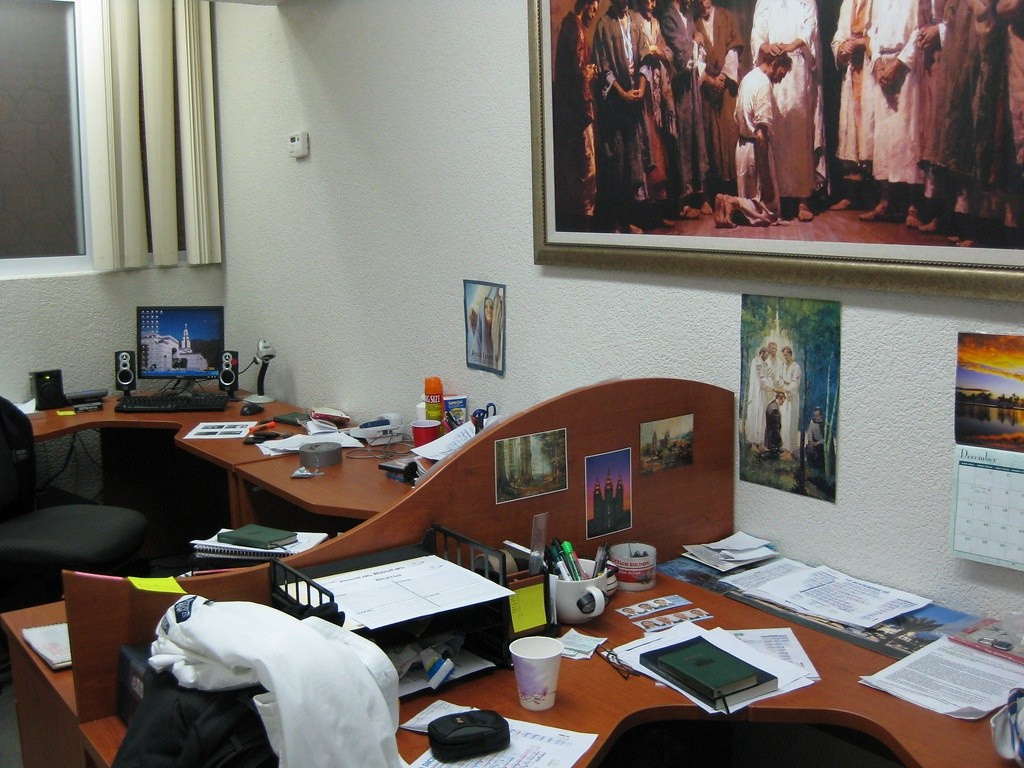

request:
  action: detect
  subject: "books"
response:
[641,634,779,711]
[190,523,328,560]
[306,418,359,435]
[22,623,72,670]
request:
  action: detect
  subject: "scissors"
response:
[473,403,496,428]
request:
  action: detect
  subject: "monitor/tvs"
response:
[135,306,225,396]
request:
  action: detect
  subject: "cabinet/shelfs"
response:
[267,522,512,704]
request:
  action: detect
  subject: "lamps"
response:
[244,338,277,405]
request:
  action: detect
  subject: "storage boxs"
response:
[420,394,466,430]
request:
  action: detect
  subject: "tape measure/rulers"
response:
[528,512,550,575]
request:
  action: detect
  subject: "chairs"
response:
[0,395,148,614]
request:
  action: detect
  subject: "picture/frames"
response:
[527,0,1024,304]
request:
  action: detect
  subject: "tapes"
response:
[472,548,517,574]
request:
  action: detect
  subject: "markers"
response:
[246,420,275,432]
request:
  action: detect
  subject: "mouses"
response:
[240,404,264,416]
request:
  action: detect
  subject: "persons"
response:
[622,596,714,631]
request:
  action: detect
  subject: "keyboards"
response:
[114,396,227,413]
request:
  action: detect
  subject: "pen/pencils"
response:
[592,542,609,577]
[470,416,476,426]
[441,412,463,432]
[545,537,590,580]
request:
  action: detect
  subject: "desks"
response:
[0,377,1024,768]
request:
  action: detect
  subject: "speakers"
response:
[27,368,66,411]
[219,350,242,402]
[115,350,136,401]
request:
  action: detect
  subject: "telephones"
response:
[349,412,405,446]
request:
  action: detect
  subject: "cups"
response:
[509,636,564,712]
[410,420,441,448]
[555,558,608,625]
